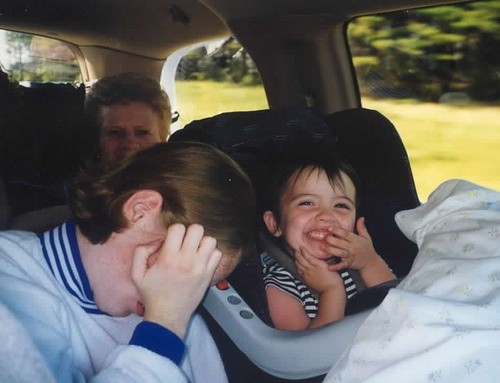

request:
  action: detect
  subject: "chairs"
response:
[167,107,420,383]
